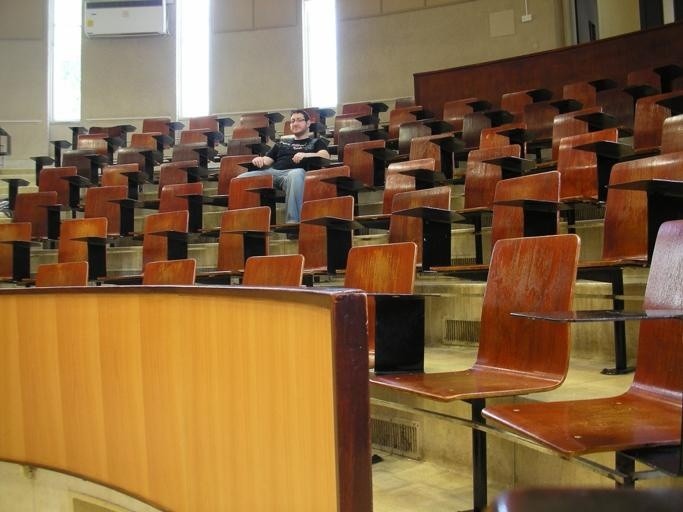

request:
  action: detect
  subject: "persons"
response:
[237,109,331,239]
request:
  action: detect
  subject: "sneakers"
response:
[286,231,297,239]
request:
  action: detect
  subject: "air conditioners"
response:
[82,0,168,40]
